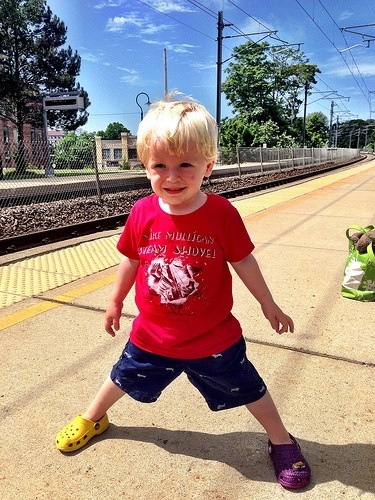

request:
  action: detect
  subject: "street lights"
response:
[136,92,152,121]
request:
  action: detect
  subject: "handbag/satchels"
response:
[341,224,375,302]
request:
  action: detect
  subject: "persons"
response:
[54,88,312,490]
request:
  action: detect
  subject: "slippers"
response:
[267,433,311,490]
[54,413,110,454]
